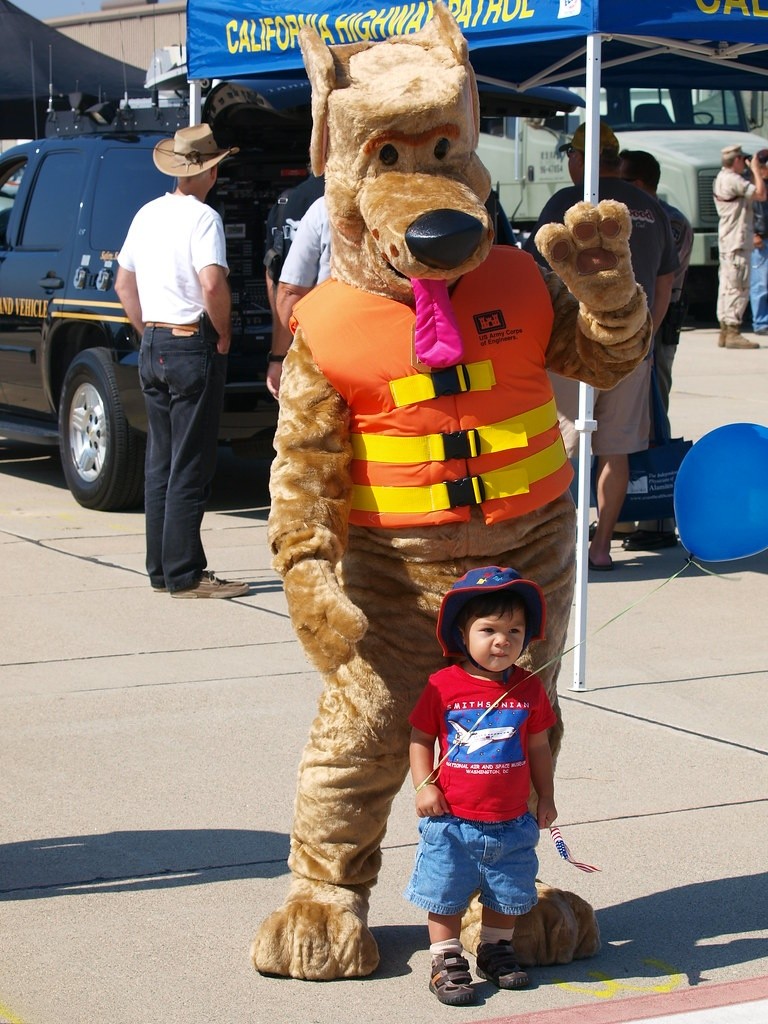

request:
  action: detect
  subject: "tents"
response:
[186,0,768,687]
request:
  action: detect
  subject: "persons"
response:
[747,163,768,336]
[589,149,695,550]
[712,144,767,349]
[522,120,680,570]
[113,123,250,598]
[409,565,559,1005]
[263,174,331,402]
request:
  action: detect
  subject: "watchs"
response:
[266,351,285,363]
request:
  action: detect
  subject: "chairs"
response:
[634,104,673,124]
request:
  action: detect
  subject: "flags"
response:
[551,828,599,873]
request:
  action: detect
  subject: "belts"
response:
[145,321,200,332]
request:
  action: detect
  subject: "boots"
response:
[718,321,728,346]
[725,322,759,349]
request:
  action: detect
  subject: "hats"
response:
[720,145,753,161]
[435,565,547,685]
[559,121,619,157]
[152,123,232,178]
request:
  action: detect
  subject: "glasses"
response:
[566,146,576,157]
[620,177,638,183]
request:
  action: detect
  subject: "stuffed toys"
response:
[252,0,654,980]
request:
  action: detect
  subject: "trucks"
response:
[474,87,768,269]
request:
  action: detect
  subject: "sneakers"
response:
[169,570,250,599]
[428,950,478,1006]
[475,938,530,989]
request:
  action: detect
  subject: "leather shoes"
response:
[587,520,640,540]
[621,529,678,551]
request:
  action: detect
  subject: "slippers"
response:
[587,547,615,571]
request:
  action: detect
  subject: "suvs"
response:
[0,76,589,513]
